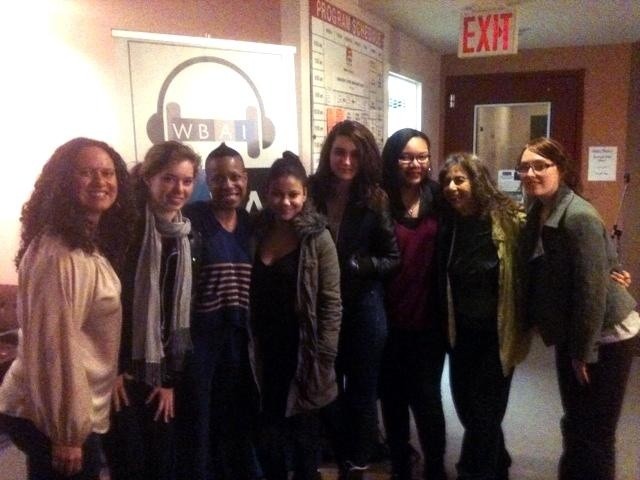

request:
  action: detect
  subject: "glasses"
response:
[516,163,557,173]
[396,153,430,163]
[442,176,470,183]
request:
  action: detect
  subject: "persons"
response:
[379,127,457,479]
[96,138,205,478]
[435,149,633,479]
[304,117,404,480]
[0,136,141,480]
[510,134,640,480]
[244,151,346,478]
[176,142,276,480]
